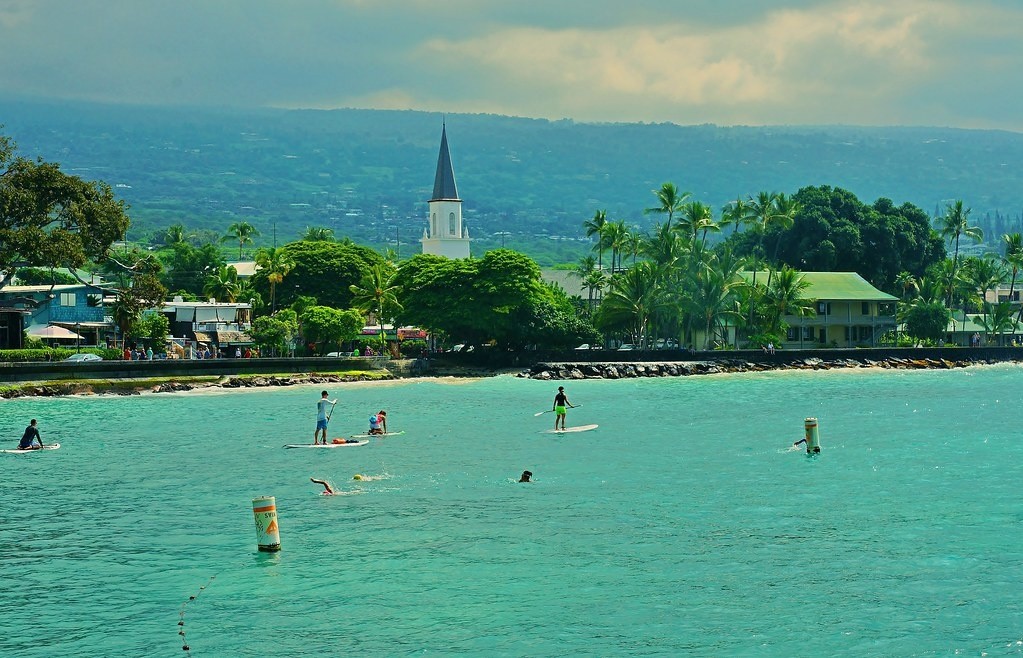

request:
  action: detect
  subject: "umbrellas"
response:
[27,325,85,344]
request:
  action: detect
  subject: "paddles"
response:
[318,401,336,445]
[533,404,584,417]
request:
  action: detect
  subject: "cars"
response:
[575,345,602,350]
[640,336,695,352]
[327,351,360,357]
[617,345,641,351]
[63,354,103,362]
[446,345,473,353]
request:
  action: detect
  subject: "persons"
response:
[519,471,532,482]
[197,346,222,359]
[553,387,574,431]
[368,411,386,435]
[308,342,316,356]
[314,391,337,445]
[689,345,696,355]
[972,331,980,347]
[124,347,154,361]
[236,347,259,358]
[17,419,45,450]
[762,341,775,355]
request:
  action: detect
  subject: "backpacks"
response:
[369,414,382,424]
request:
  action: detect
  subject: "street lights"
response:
[76,322,81,354]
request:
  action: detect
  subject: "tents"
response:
[397,324,426,341]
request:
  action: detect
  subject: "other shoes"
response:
[562,428,566,430]
[555,429,559,431]
[314,442,321,445]
[368,430,371,435]
[323,441,329,445]
[372,430,377,435]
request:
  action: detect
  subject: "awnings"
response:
[362,324,397,335]
[193,331,214,342]
[217,331,256,347]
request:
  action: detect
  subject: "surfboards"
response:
[538,424,599,434]
[285,439,370,448]
[0,443,60,453]
[352,431,407,438]
